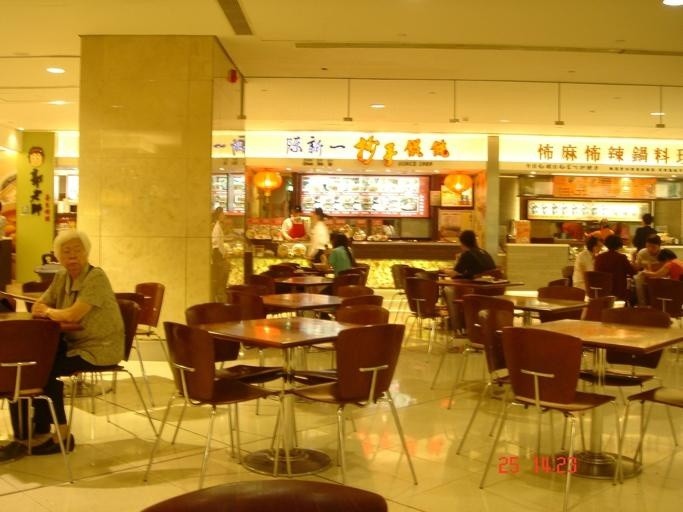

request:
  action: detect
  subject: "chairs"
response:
[387,264,410,322]
[61,299,158,455]
[648,278,683,315]
[310,305,390,431]
[578,306,679,463]
[141,478,388,512]
[570,295,616,379]
[454,308,570,472]
[168,303,287,459]
[444,294,515,409]
[223,290,263,303]
[480,327,624,512]
[581,270,613,300]
[243,272,276,295]
[403,277,449,353]
[535,286,585,324]
[110,284,170,394]
[611,385,683,486]
[273,325,419,489]
[141,321,290,489]
[334,286,373,297]
[332,273,361,286]
[0,319,74,485]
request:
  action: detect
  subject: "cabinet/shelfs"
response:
[506,243,569,290]
[243,240,462,286]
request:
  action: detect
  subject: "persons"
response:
[0,201,7,237]
[641,248,683,280]
[637,234,662,269]
[325,231,353,277]
[0,229,125,455]
[572,237,603,295]
[211,206,225,263]
[632,214,657,252]
[443,230,497,279]
[309,207,329,264]
[281,205,309,242]
[594,235,638,304]
[556,219,615,244]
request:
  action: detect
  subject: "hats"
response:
[294,206,301,213]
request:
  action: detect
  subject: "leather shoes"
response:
[31,433,75,455]
